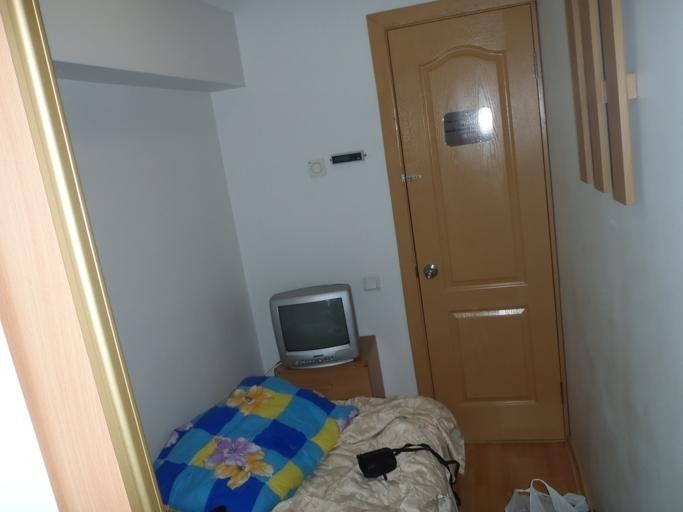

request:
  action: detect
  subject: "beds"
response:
[152,398,462,508]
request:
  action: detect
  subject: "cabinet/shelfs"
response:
[275,334,385,401]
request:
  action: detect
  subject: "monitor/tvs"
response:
[268,283,361,370]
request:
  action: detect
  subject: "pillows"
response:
[152,377,357,510]
